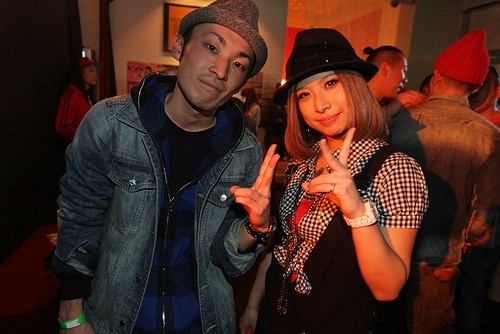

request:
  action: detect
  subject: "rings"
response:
[330,183,335,194]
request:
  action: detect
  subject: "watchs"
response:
[245,215,277,241]
[342,196,379,229]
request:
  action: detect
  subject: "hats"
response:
[435,29,491,88]
[273,27,379,105]
[176,0,268,79]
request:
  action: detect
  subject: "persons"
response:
[452,65,499,334]
[56,56,99,156]
[52,0,268,334]
[240,87,261,136]
[419,74,432,97]
[385,28,500,334]
[363,46,425,121]
[237,28,428,334]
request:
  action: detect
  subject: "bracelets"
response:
[57,310,87,329]
